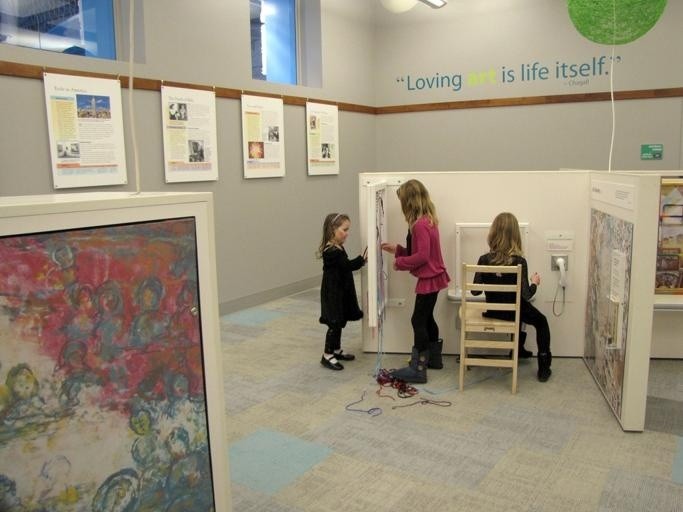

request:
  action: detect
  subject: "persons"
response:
[380,178,451,384]
[471,211,552,382]
[314,212,368,371]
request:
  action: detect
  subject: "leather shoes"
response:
[333,349,355,361]
[320,354,344,370]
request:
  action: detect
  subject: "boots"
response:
[536,351,552,382]
[390,346,429,384]
[426,338,444,370]
[510,332,533,358]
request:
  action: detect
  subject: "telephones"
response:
[555,257,568,288]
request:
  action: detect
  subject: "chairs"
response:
[458,263,522,395]
